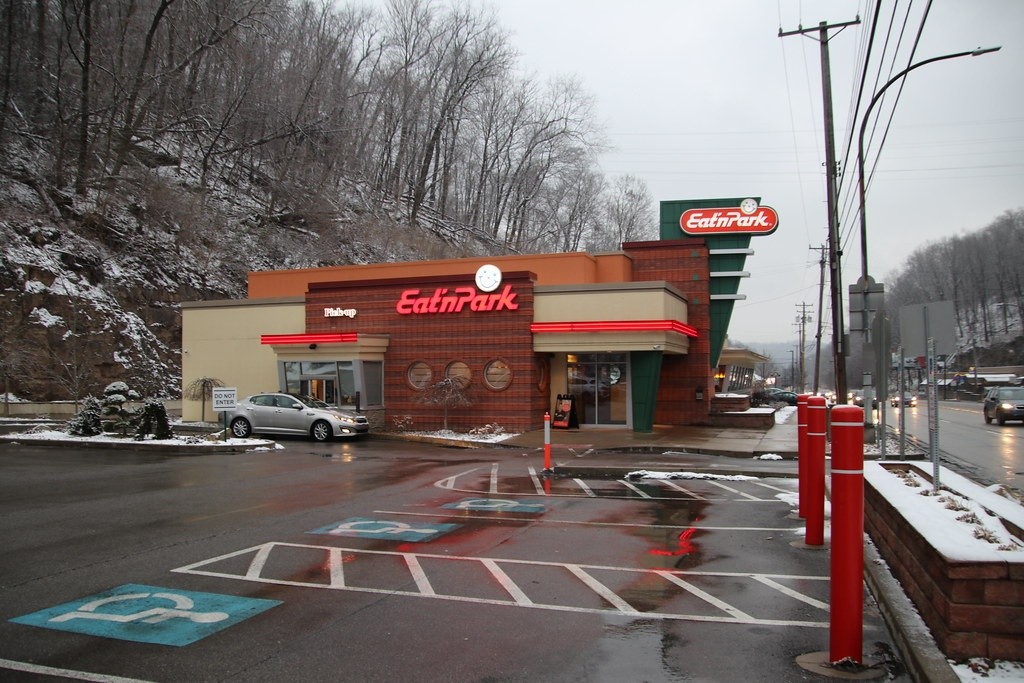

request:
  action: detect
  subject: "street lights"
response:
[786,349,795,391]
[857,44,1002,442]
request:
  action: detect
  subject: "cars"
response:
[765,391,798,406]
[220,392,370,442]
[820,389,853,403]
[891,391,917,407]
[752,387,785,399]
[982,386,1024,424]
[852,390,878,409]
